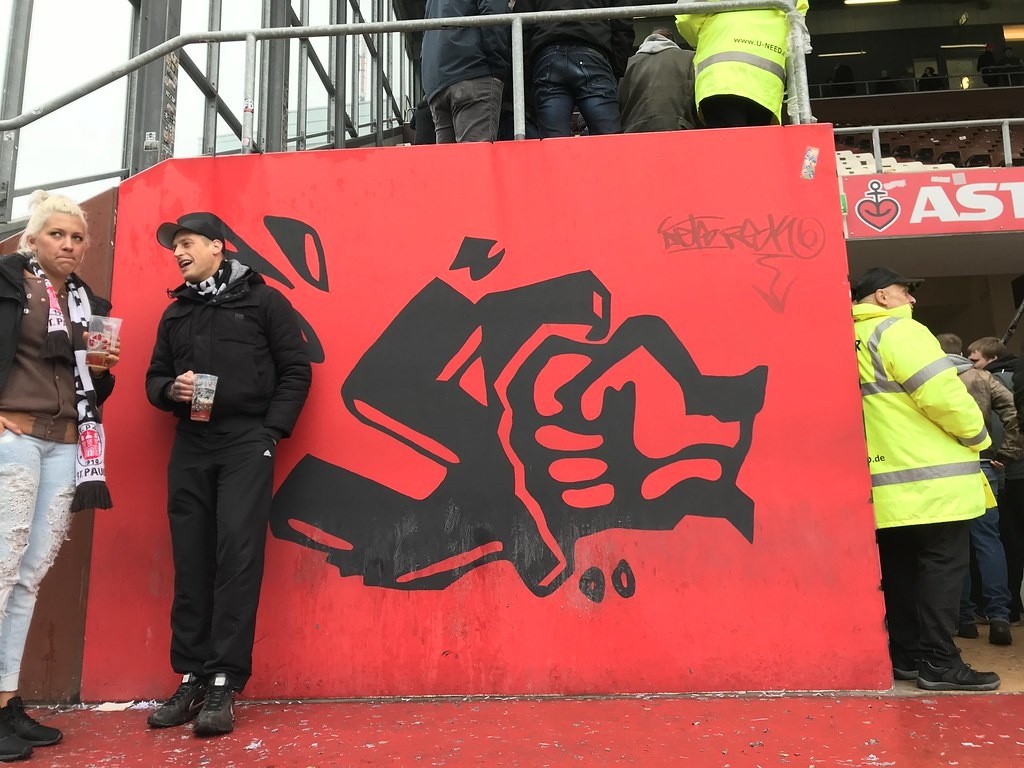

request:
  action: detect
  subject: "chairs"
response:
[833,118,1024,175]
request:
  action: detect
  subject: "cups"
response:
[85,315,123,370]
[190,373,218,422]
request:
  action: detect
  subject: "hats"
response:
[155,218,226,251]
[851,268,924,303]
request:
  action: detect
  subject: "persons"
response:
[422,0,511,144]
[674,0,810,128]
[410,95,435,145]
[977,44,1024,87]
[828,64,855,95]
[618,28,699,132]
[918,67,938,91]
[0,190,120,761]
[144,221,312,732]
[512,0,635,139]
[851,267,1001,690]
[936,334,1024,646]
[577,113,589,135]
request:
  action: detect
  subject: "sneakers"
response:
[2,696,64,745]
[958,623,979,638]
[917,659,1002,691]
[0,719,33,760]
[892,661,921,680]
[193,672,235,734]
[147,672,203,728]
[989,621,1013,646]
